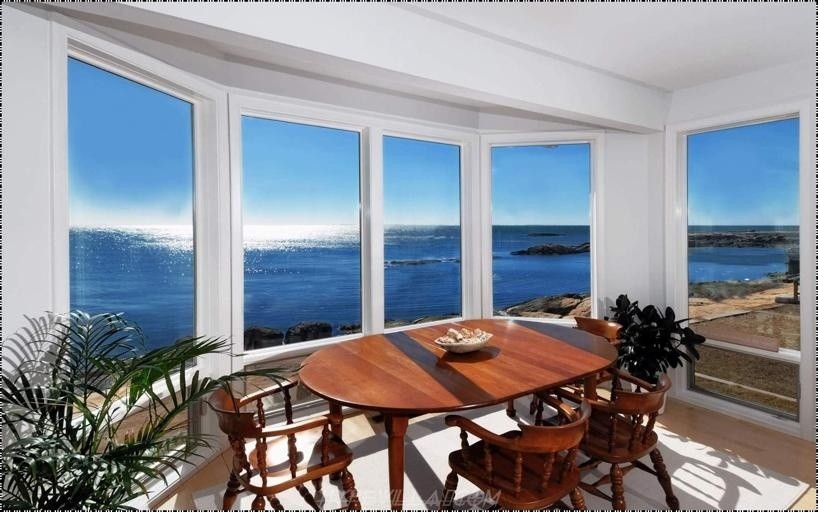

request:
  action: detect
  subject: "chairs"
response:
[209,375,364,511]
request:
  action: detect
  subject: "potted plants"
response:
[603,293,706,414]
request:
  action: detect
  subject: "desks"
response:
[297,317,619,510]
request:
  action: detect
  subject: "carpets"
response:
[190,392,813,511]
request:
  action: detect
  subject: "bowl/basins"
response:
[433,332,493,354]
